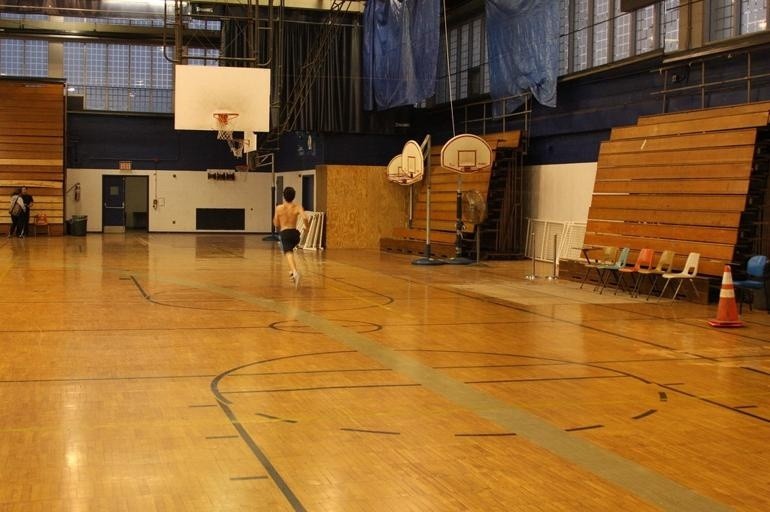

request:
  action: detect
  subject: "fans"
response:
[461,189,490,267]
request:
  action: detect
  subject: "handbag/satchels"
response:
[9,196,23,217]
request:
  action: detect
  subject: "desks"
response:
[571,244,606,288]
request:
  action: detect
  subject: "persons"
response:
[272,187,309,290]
[18,186,33,237]
[8,187,26,237]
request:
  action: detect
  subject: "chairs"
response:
[657,252,700,306]
[613,248,656,298]
[580,246,620,291]
[733,256,770,315]
[634,250,680,300]
[597,247,630,295]
[34,214,50,242]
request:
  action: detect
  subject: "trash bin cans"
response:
[133,212,146,228]
[71,215,87,235]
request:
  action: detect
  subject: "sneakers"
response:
[289,271,301,292]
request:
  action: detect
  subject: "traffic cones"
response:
[708,263,747,328]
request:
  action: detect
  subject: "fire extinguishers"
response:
[74,183,80,201]
